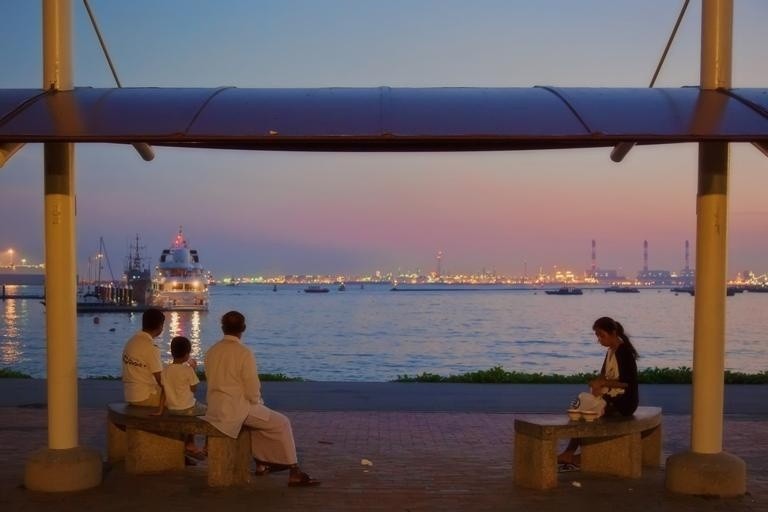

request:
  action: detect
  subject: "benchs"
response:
[510,404,664,490]
[107,399,254,486]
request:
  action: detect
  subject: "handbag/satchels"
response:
[569,391,608,420]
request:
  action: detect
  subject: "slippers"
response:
[254,464,291,475]
[557,463,582,474]
[288,471,323,488]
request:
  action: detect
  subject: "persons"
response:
[149,336,209,460]
[195,309,322,487]
[122,309,198,467]
[556,316,643,473]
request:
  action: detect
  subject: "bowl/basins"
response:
[567,408,597,422]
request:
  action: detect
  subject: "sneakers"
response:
[185,447,209,467]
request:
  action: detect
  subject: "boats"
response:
[38,225,211,314]
[545,286,584,295]
[304,284,330,293]
[272,284,278,292]
[605,286,640,293]
[671,283,768,297]
[339,283,346,292]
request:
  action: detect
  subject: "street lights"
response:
[8,248,14,265]
[21,258,26,265]
